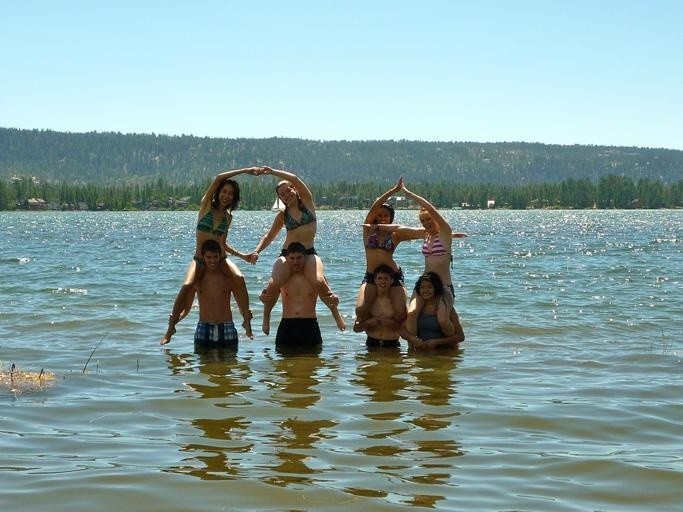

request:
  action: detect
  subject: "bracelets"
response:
[231,248,238,255]
[269,168,273,176]
[376,224,380,230]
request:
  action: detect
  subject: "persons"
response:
[258,242,337,349]
[354,176,470,332]
[360,176,462,353]
[167,238,253,349]
[353,263,405,348]
[159,166,259,346]
[409,360,459,504]
[263,356,332,487]
[249,163,347,335]
[170,355,250,481]
[396,271,464,355]
[349,357,409,500]
